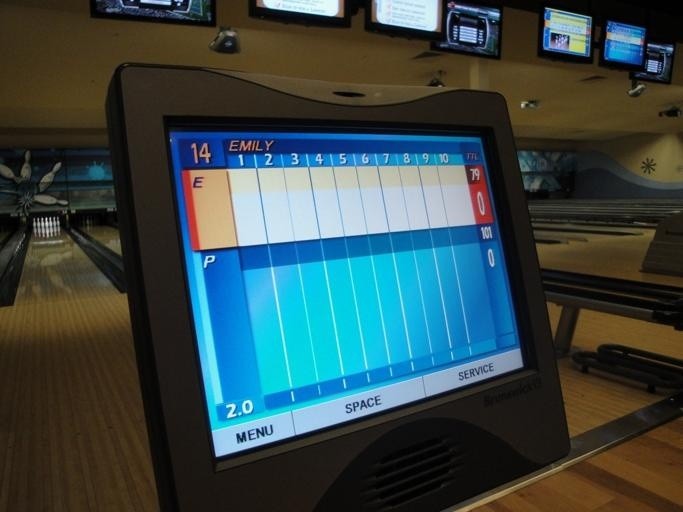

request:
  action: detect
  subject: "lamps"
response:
[209,26,241,54]
[627,77,647,97]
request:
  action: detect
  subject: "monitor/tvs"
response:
[629,39,675,85]
[248,0,352,28]
[365,0,446,39]
[598,18,649,70]
[105,61,570,512]
[538,6,595,65]
[430,0,502,59]
[90,0,216,26]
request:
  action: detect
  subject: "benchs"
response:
[541,267,683,352]
